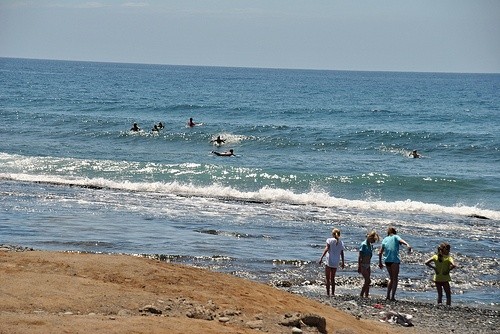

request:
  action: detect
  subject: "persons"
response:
[378,228,412,301]
[425,244,456,307]
[357,230,380,298]
[152,125,159,132]
[130,122,140,131]
[187,118,195,126]
[409,150,421,159]
[158,122,164,129]
[319,228,345,297]
[212,148,236,156]
[210,135,225,144]
